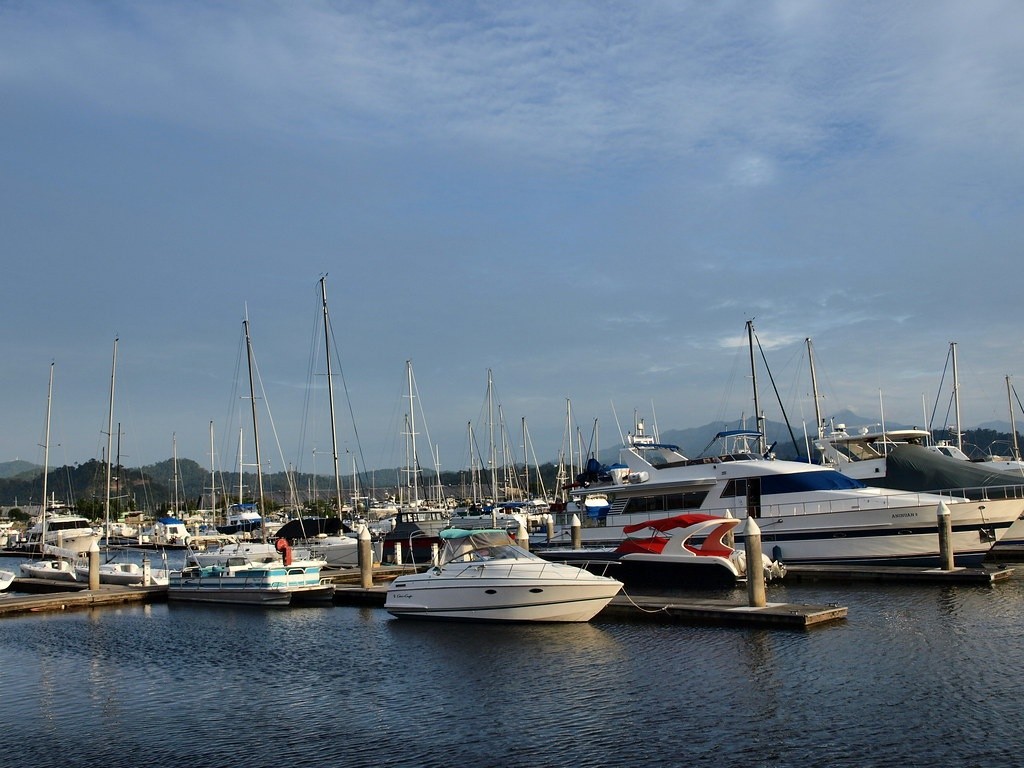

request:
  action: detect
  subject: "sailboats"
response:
[0,271,1024,605]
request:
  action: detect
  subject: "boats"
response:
[384,527,626,622]
